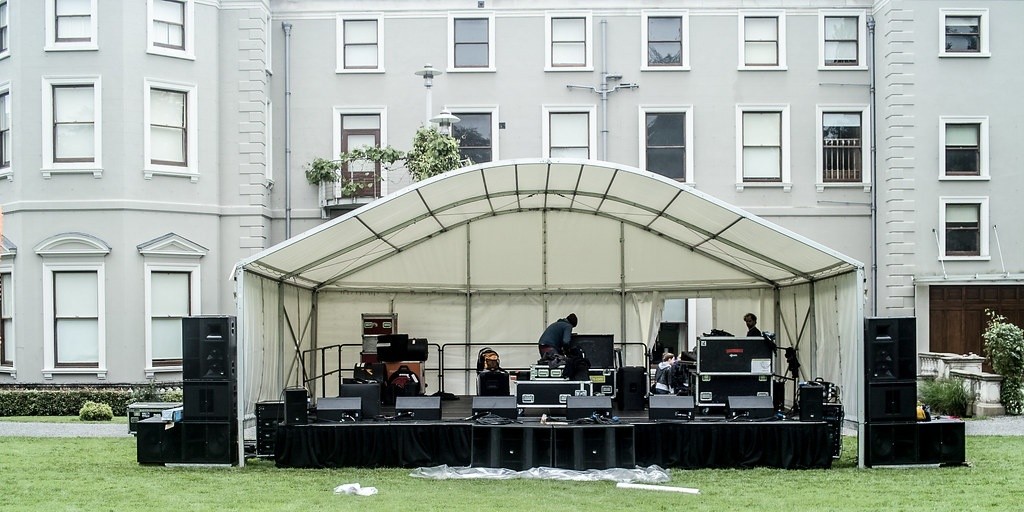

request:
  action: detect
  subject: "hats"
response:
[566,313,577,327]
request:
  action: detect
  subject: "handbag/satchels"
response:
[651,384,656,394]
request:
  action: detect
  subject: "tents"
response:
[235,157,865,472]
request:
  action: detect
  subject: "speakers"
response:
[566,396,612,421]
[136,316,237,464]
[395,396,442,419]
[342,384,381,419]
[864,316,965,468]
[649,396,694,420]
[285,388,308,425]
[471,396,517,420]
[800,385,823,422]
[616,366,645,412]
[725,396,774,420]
[317,397,362,420]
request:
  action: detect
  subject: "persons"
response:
[743,313,761,336]
[654,353,675,394]
[562,346,591,381]
[539,313,578,358]
